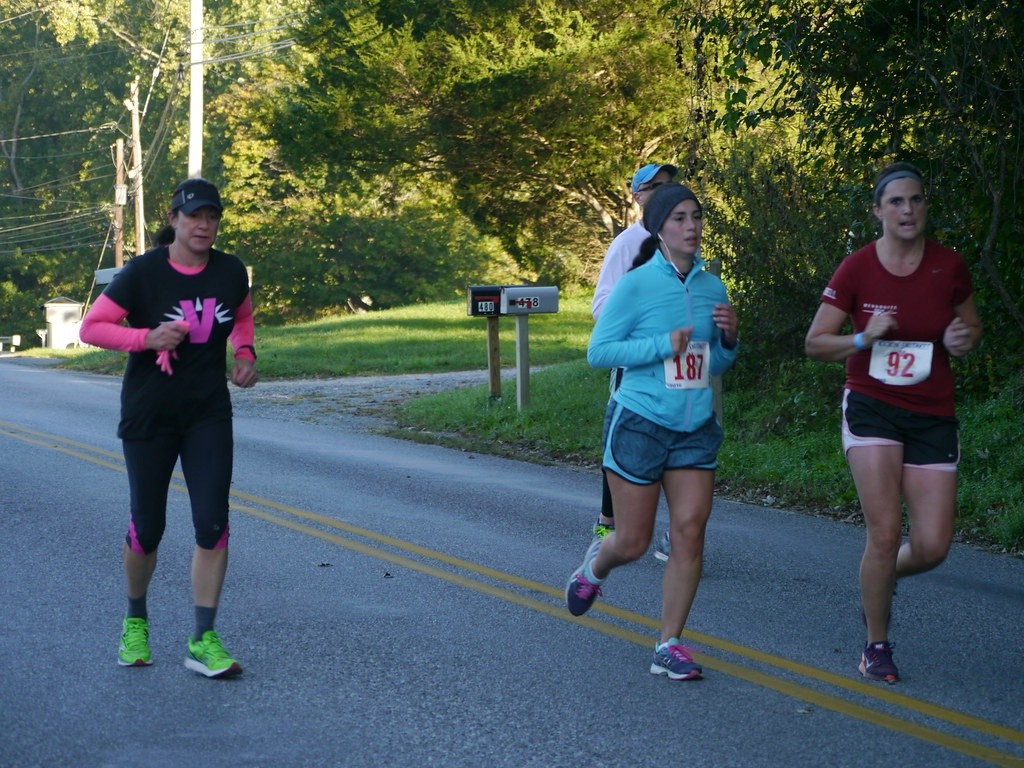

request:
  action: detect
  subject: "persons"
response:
[81,178,258,679]
[565,183,739,682]
[592,163,680,562]
[803,162,984,683]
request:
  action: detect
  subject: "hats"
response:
[631,164,678,193]
[643,183,702,243]
[172,178,223,216]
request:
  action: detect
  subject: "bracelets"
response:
[854,333,864,350]
[236,345,257,361]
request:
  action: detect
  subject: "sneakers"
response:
[183,630,243,678]
[653,531,704,577]
[594,514,616,541]
[118,612,153,666]
[859,640,900,681]
[860,580,898,634]
[650,637,704,680]
[565,539,611,617]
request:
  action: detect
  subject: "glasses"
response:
[638,181,672,192]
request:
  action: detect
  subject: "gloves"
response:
[155,319,191,376]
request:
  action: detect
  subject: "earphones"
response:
[657,233,664,241]
